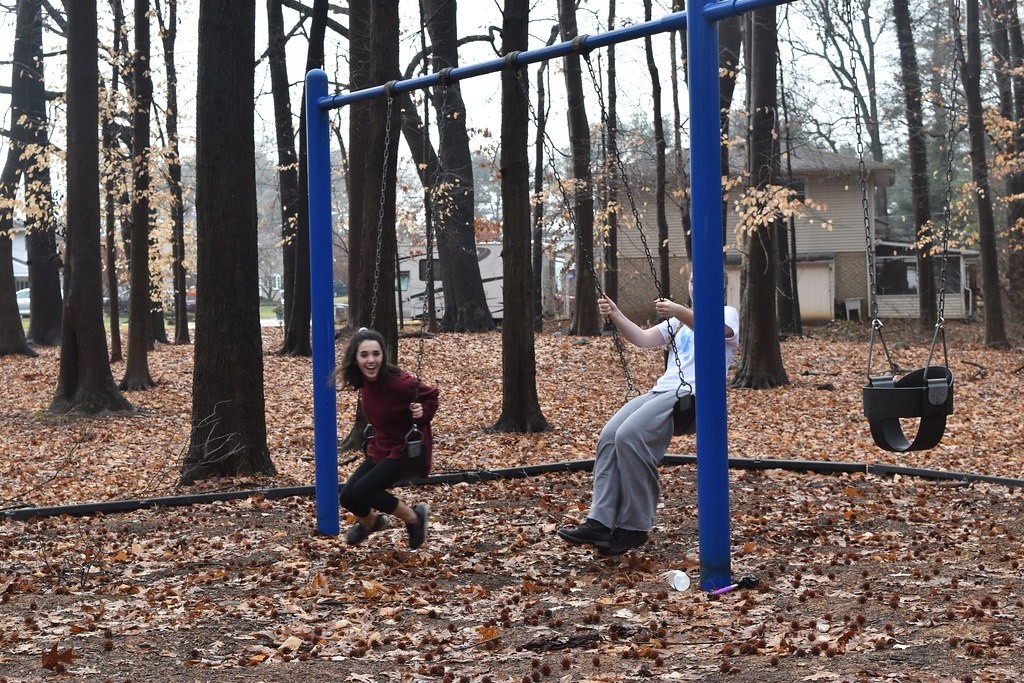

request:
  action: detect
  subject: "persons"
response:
[556,269,740,555]
[339,331,439,550]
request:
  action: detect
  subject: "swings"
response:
[844,0,964,454]
[504,33,698,438]
[357,64,453,490]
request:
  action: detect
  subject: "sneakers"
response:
[557,518,612,549]
[598,527,649,555]
[346,514,388,546]
[405,502,428,550]
